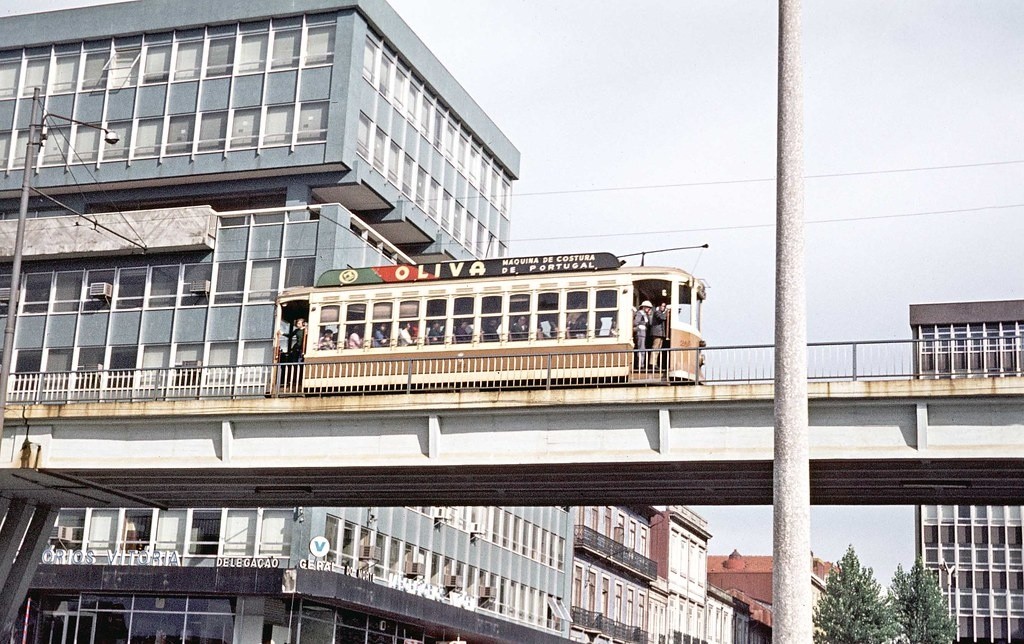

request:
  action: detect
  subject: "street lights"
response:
[939,559,955,644]
[0,114,119,443]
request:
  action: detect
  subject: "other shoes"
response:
[650,365,659,369]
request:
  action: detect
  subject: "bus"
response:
[266,252,707,398]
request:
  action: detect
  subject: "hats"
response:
[639,301,653,308]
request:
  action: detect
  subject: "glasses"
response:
[520,319,525,321]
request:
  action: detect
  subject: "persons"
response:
[318,302,618,350]
[277,318,307,385]
[633,301,671,370]
